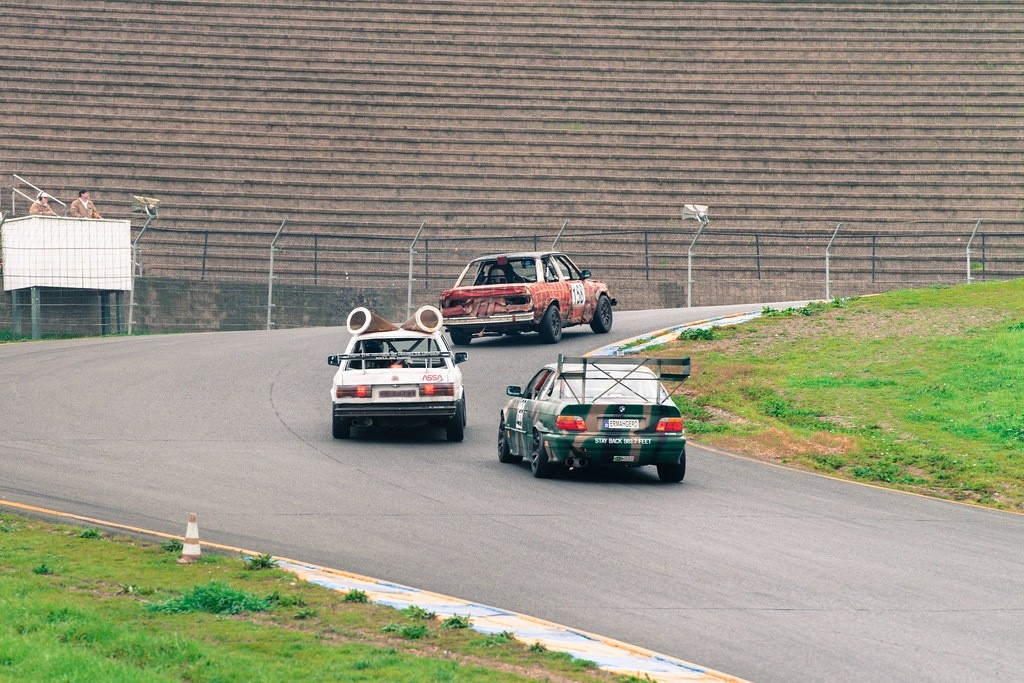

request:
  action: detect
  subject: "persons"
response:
[70,188,104,219]
[30,191,58,216]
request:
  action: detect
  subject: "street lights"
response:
[681,203,710,308]
[125,195,161,334]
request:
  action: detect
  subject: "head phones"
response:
[38,192,43,201]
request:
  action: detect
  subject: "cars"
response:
[496,361,688,483]
[327,328,469,443]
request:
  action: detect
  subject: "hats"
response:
[37,192,50,199]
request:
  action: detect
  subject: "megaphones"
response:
[682,205,710,220]
[132,196,160,215]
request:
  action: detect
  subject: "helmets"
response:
[364,340,385,353]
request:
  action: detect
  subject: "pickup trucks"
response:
[438,250,618,347]
